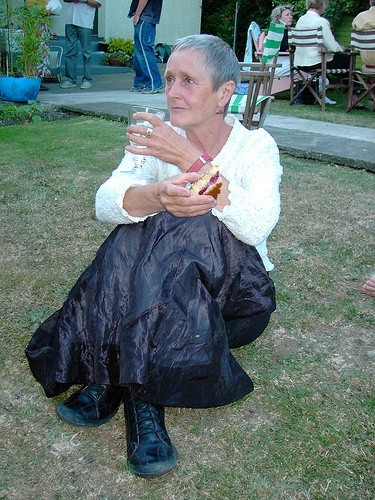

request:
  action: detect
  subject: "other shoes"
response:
[321,96,337,105]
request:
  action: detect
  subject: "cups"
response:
[127,105,166,149]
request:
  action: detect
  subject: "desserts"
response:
[189,166,222,200]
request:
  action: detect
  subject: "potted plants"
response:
[0,0,53,102]
[105,51,131,66]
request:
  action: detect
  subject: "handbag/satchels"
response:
[295,82,315,105]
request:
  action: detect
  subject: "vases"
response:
[98,42,114,53]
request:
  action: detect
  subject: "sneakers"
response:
[130,86,144,92]
[61,81,77,87]
[143,86,164,94]
[81,82,91,89]
[55,382,122,426]
[120,382,177,477]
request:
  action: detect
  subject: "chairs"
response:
[245,20,375,113]
[40,46,64,83]
[227,22,286,131]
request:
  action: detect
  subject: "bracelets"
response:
[186,154,211,173]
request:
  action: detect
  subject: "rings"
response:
[146,128,153,137]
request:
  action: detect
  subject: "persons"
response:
[24,0,56,91]
[25,34,283,478]
[127,0,163,94]
[61,0,102,89]
[293,0,363,108]
[256,5,337,104]
[352,0,375,73]
